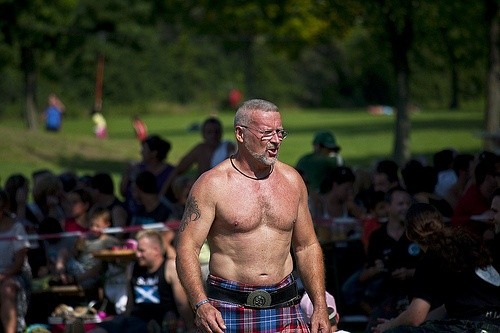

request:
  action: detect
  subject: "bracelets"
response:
[193,299,210,313]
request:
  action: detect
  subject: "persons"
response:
[1,116,500,333]
[173,99,330,333]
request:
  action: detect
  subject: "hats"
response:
[313,131,342,150]
[300,290,336,323]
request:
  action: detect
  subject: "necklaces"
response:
[229,154,273,180]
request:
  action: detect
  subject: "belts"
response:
[204,279,299,307]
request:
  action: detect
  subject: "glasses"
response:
[239,124,288,140]
